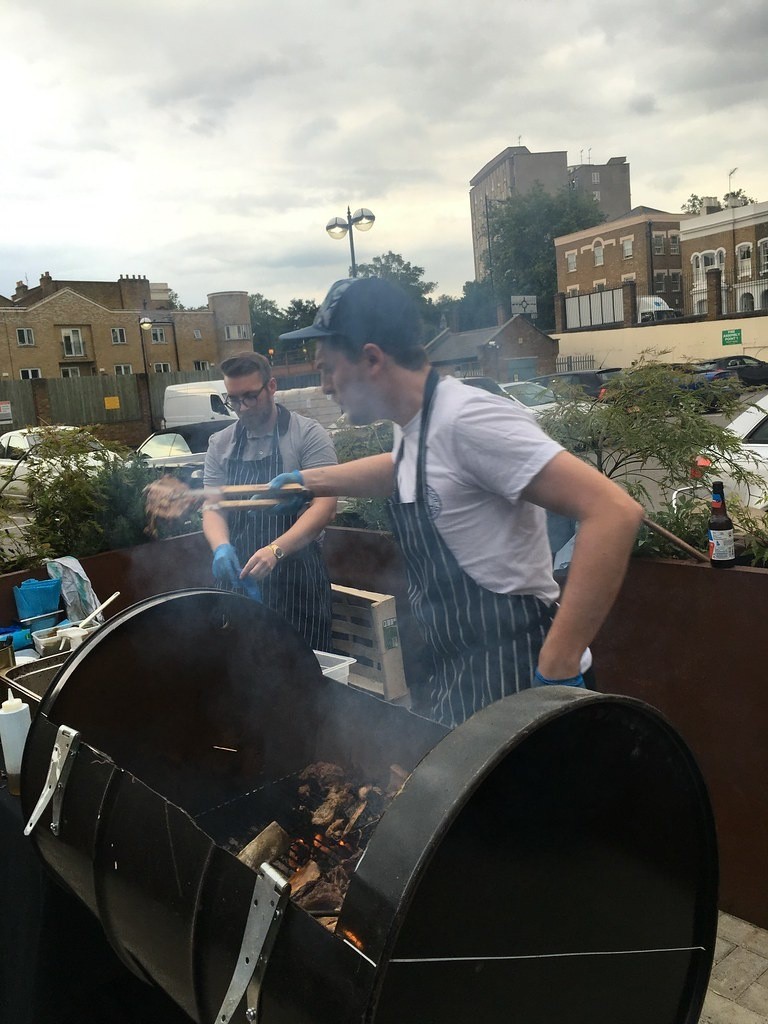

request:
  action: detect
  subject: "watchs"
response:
[268,544,285,560]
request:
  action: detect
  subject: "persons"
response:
[254,277,645,726]
[201,353,336,656]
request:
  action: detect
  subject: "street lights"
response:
[325,205,375,281]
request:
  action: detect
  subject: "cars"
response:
[672,394,768,515]
[526,367,637,413]
[125,419,240,488]
[698,356,768,389]
[497,382,610,421]
[326,373,503,434]
[662,360,740,414]
[0,425,125,504]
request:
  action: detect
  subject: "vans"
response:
[160,382,239,426]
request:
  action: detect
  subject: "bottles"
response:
[0,687,32,796]
[707,481,736,568]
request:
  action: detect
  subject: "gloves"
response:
[237,570,264,603]
[210,543,242,581]
[532,669,584,689]
[246,470,308,518]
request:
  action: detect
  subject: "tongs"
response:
[169,483,314,512]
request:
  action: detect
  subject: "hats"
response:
[278,276,423,349]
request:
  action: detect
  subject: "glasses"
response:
[224,381,266,411]
